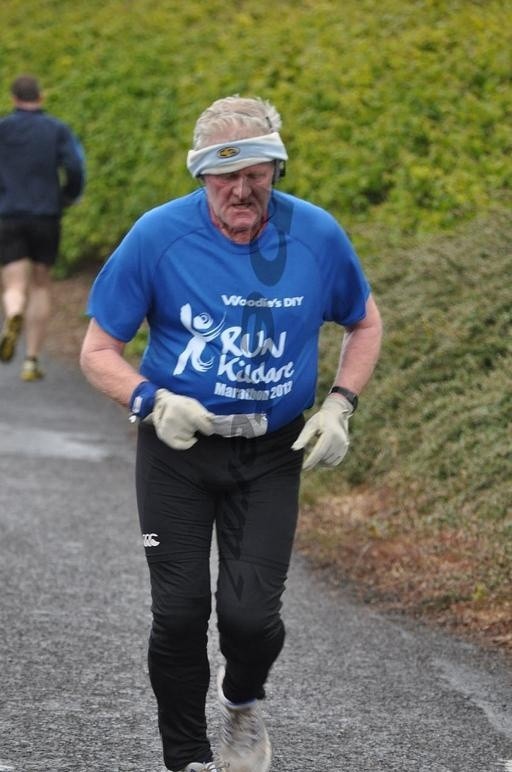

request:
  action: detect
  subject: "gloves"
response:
[290,394,354,471]
[141,388,216,451]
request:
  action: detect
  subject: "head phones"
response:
[266,116,286,185]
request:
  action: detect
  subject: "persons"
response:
[75,92,384,771]
[0,75,85,384]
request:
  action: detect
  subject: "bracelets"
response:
[327,385,359,413]
[128,379,163,421]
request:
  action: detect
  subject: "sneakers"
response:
[20,369,43,381]
[1,315,25,362]
[177,761,218,772]
[217,664,270,772]
